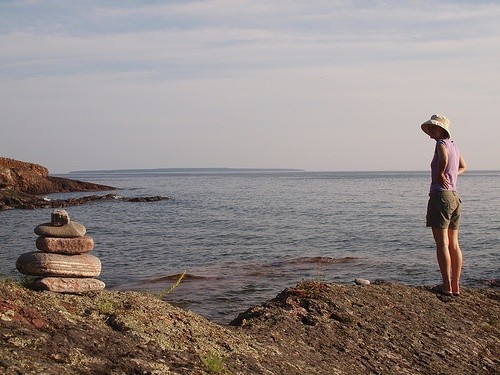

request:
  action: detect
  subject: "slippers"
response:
[438,284,461,296]
[432,287,453,296]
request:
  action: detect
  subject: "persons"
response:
[420,114,468,296]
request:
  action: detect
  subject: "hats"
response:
[421,114,451,140]
